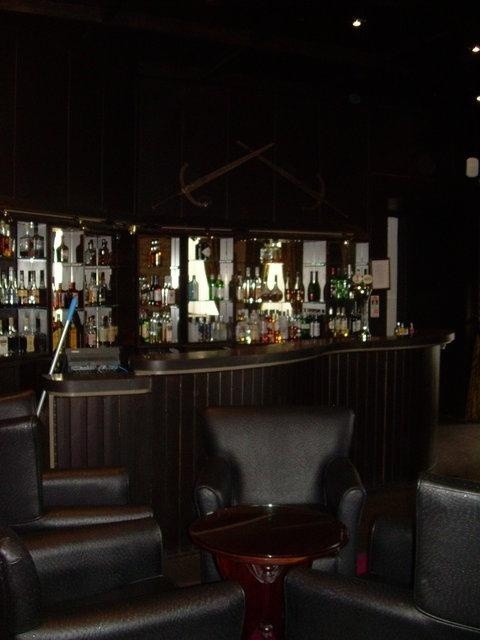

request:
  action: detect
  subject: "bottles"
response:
[395,321,413,338]
[147,240,162,268]
[137,264,373,347]
[260,242,281,264]
[0,219,118,359]
[196,240,209,261]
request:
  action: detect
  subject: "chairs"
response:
[0,518,248,640]
[191,405,366,576]
[285,472,480,639]
[0,388,154,534]
